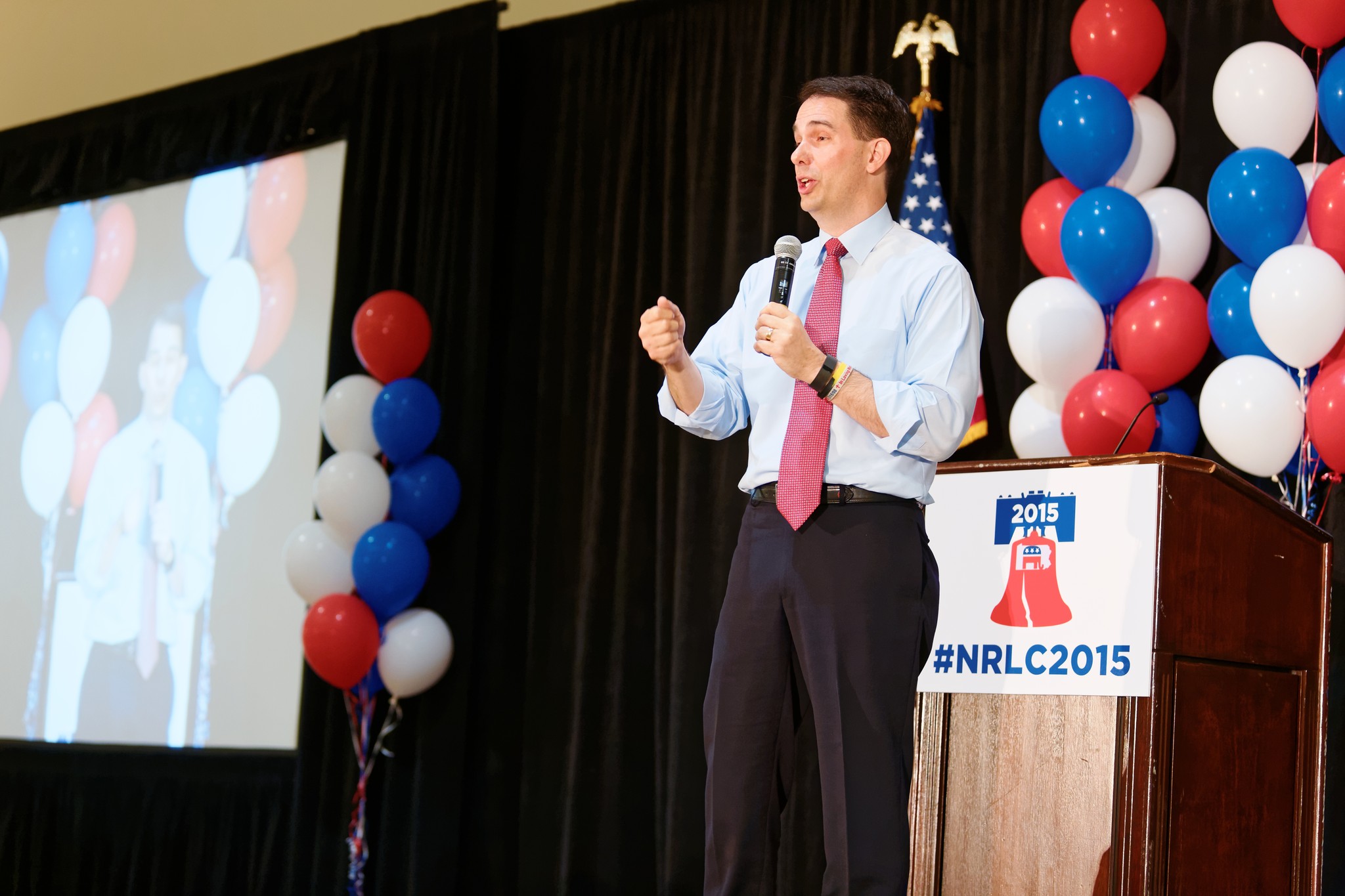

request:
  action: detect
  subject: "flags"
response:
[893,98,987,449]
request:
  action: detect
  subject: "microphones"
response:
[1114,393,1169,455]
[764,235,802,357]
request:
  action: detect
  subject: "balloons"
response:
[319,375,381,459]
[352,522,431,622]
[286,518,356,605]
[381,611,453,698]
[386,459,461,538]
[315,452,392,551]
[0,150,310,514]
[369,378,443,469]
[303,594,378,697]
[1003,0,1345,481]
[352,288,434,384]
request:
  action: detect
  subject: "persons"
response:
[73,306,212,746]
[639,76,985,896]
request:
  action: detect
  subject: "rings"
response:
[765,327,774,341]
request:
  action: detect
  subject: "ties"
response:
[778,238,849,531]
[136,438,160,681]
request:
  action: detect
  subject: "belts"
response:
[749,483,925,516]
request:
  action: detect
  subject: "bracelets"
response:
[808,353,854,401]
